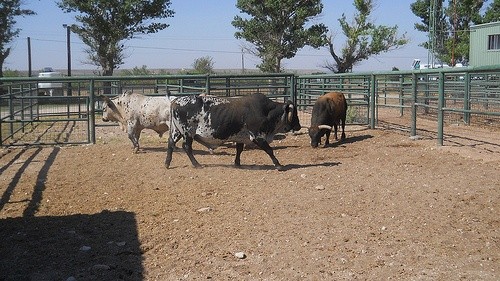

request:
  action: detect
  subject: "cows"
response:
[100,90,213,155]
[164,93,301,171]
[300,92,347,148]
